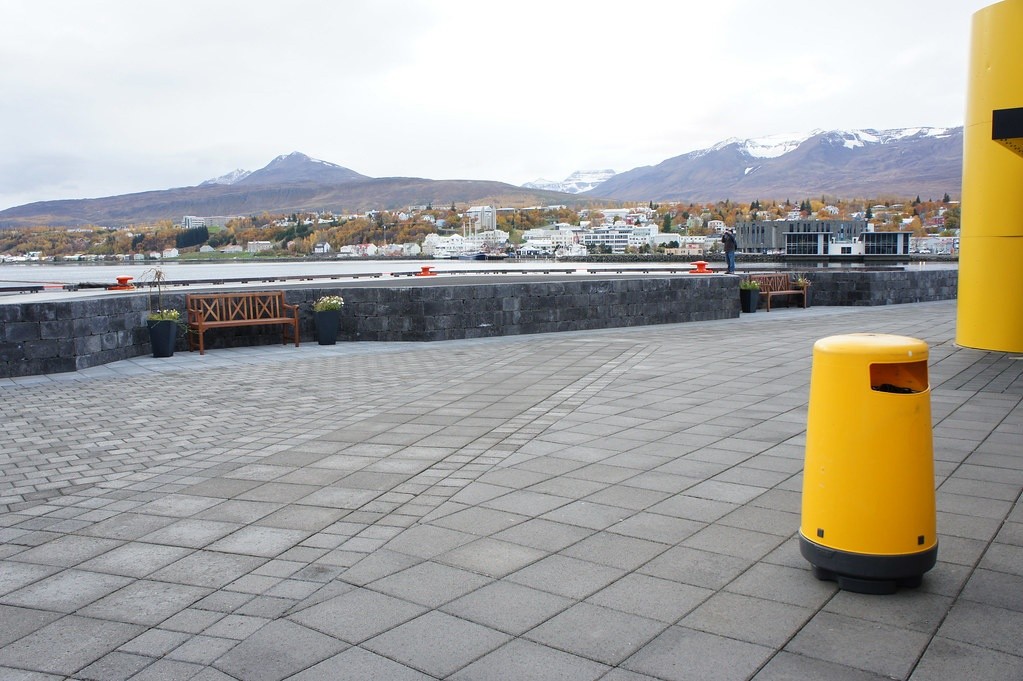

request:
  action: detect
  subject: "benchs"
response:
[185,293,299,355]
[751,273,807,312]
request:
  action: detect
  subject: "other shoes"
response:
[725,270,733,274]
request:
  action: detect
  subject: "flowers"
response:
[794,275,812,287]
[740,276,763,290]
[309,295,344,312]
[146,308,201,335]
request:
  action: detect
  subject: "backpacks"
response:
[725,235,737,250]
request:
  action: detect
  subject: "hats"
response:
[726,229,734,234]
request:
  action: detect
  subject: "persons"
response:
[721,228,737,274]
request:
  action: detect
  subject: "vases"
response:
[148,320,179,357]
[741,289,760,312]
[796,286,812,306]
[312,310,340,345]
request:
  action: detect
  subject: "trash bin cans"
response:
[799,333,940,597]
